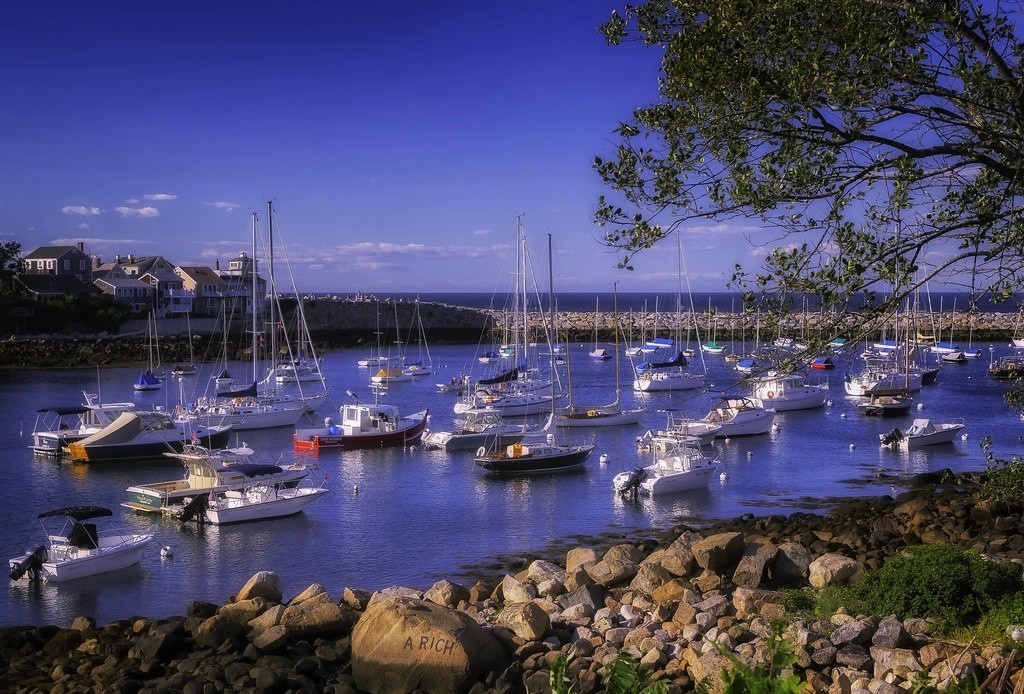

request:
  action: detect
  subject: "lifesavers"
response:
[767,391,774,398]
[477,446,485,456]
[644,373,650,379]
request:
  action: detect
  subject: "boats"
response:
[120,405,321,515]
[7,504,155,584]
[679,394,780,437]
[614,429,720,497]
[878,416,965,451]
[160,473,329,529]
[422,397,526,454]
[295,380,432,450]
[634,414,724,455]
[62,370,246,465]
[27,345,200,458]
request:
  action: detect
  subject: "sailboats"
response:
[165,196,330,431]
[545,277,649,427]
[357,296,437,383]
[473,230,597,470]
[134,311,164,391]
[592,228,1024,397]
[455,211,573,418]
[856,283,912,417]
[742,281,830,412]
